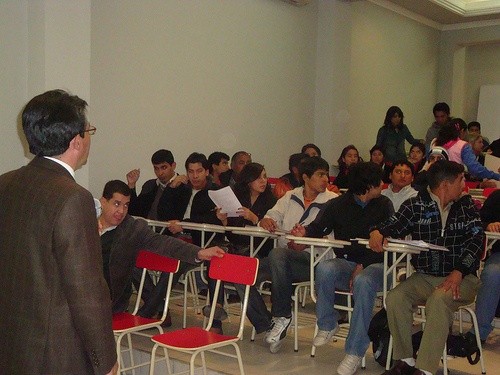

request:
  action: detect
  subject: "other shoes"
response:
[201,304,228,321]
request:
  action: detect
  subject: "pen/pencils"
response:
[382,234,385,246]
[301,221,305,226]
[272,220,278,227]
[211,209,216,211]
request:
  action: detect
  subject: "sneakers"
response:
[205,320,224,335]
[264,317,294,343]
[157,310,172,327]
[381,361,426,375]
[270,338,285,353]
[313,325,340,347]
[336,354,361,375]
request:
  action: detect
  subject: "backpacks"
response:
[446,333,481,365]
[367,309,392,368]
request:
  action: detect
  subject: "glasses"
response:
[85,125,96,135]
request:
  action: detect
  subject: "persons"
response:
[0,89,500,375]
[235,156,338,355]
[132,152,223,318]
[96,180,226,317]
[470,169,500,343]
[126,149,194,239]
[0,89,118,375]
[368,158,486,375]
[201,163,276,333]
[289,162,395,375]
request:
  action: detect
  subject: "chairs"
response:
[150,251,260,375]
[130,215,485,375]
[112,248,182,375]
